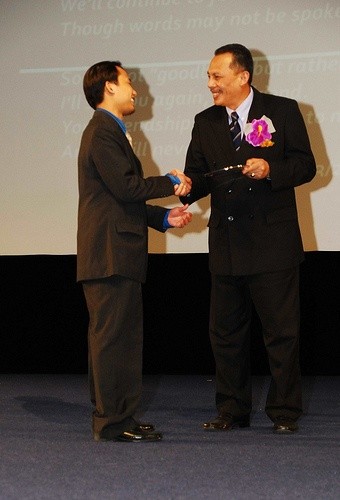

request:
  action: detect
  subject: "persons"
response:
[76,62,192,442]
[174,43,316,434]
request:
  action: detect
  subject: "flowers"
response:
[243,116,278,148]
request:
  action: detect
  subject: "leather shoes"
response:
[203,416,251,432]
[137,424,155,430]
[111,430,163,443]
[274,422,299,434]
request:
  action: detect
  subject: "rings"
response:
[251,173,255,177]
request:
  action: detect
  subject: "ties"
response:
[228,112,243,151]
